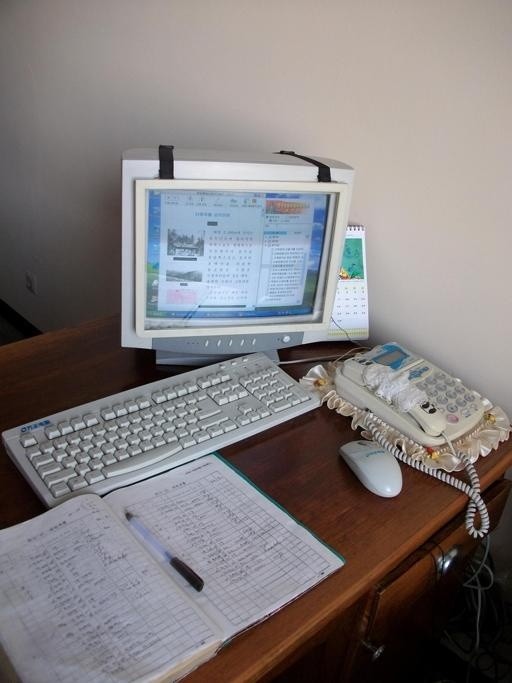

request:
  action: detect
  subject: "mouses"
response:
[339,440,403,497]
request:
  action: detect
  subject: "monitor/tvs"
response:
[119,144,355,364]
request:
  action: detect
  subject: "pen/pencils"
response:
[124,511,205,593]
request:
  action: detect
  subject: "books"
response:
[0,454,348,682]
[327,224,370,342]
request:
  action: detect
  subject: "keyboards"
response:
[2,353,323,509]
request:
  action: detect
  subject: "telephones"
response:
[334,340,485,448]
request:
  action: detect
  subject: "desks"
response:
[1,309,510,683]
